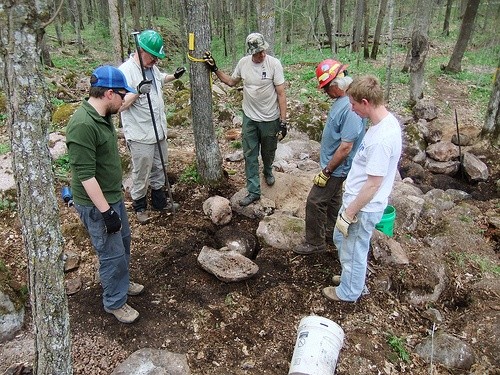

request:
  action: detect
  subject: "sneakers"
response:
[127,281,144,295]
[264,170,275,186]
[239,193,259,206]
[104,303,140,324]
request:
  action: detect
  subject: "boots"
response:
[150,187,179,211]
[133,198,150,225]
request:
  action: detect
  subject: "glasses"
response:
[107,88,126,100]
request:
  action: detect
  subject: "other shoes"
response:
[327,244,337,252]
[332,275,341,285]
[293,242,326,254]
[322,287,343,301]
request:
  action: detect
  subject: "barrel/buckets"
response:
[288,316,346,375]
[375,204,397,239]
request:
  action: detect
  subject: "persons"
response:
[322,75,402,301]
[66,66,145,323]
[203,33,287,205]
[294,58,366,254]
[118,30,187,223]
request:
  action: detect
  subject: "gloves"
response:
[336,208,358,238]
[275,120,287,142]
[100,206,122,233]
[202,51,218,72]
[174,67,187,79]
[313,167,332,188]
[137,79,153,95]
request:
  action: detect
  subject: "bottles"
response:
[62,185,72,203]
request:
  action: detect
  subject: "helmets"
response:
[315,59,348,89]
[137,30,165,59]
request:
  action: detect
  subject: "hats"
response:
[90,65,136,94]
[246,33,269,54]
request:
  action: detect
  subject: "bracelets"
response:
[324,167,332,175]
